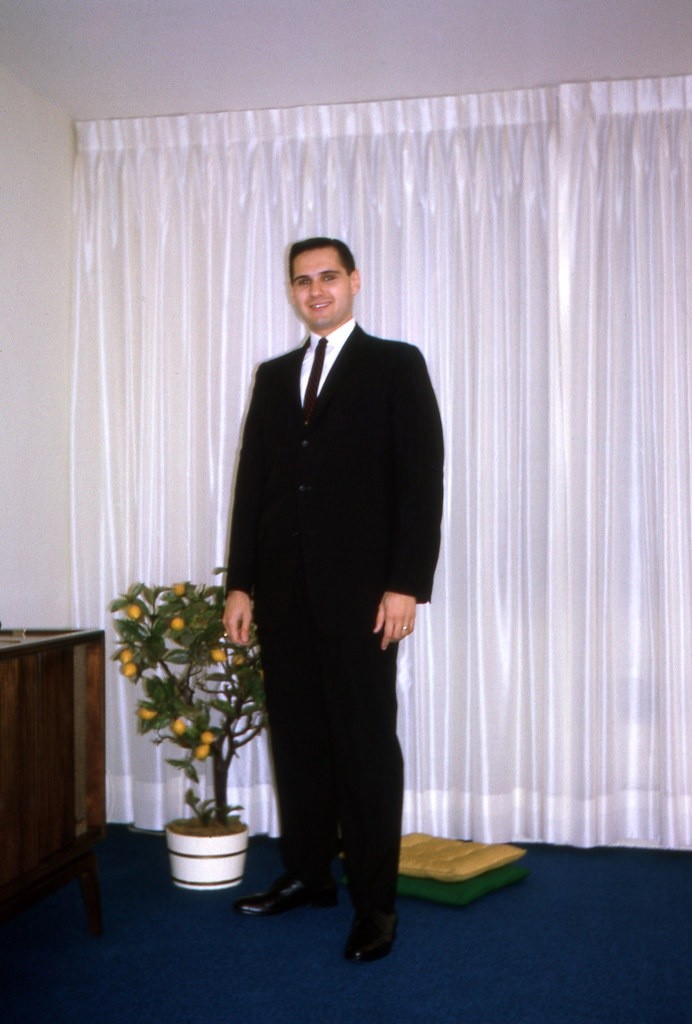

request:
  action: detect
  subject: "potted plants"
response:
[111,569,273,892]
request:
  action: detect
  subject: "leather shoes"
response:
[342,905,399,964]
[233,865,342,916]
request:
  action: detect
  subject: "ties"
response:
[302,336,329,428]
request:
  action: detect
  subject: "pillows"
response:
[339,832,529,906]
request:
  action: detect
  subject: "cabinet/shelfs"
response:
[0,625,106,936]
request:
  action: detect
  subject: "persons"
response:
[218,235,444,965]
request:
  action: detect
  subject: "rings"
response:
[401,625,411,630]
